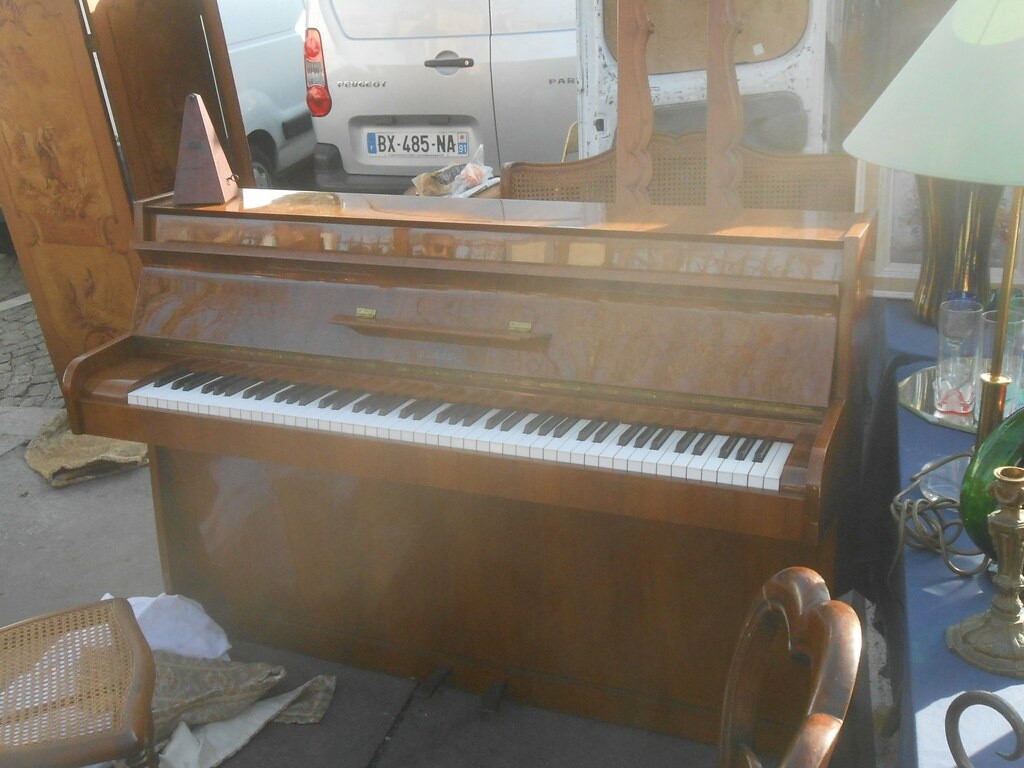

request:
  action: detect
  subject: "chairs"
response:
[0,596,155,768]
[718,566,862,768]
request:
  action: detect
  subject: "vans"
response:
[79,0,305,193]
[306,2,578,192]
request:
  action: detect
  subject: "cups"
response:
[975,310,1024,424]
[1009,296,1024,312]
[934,299,983,414]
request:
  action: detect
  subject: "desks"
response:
[894,360,1024,768]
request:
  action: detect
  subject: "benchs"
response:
[501,133,857,212]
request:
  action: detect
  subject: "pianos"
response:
[62,186,879,747]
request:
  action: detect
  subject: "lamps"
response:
[843,0,1024,452]
